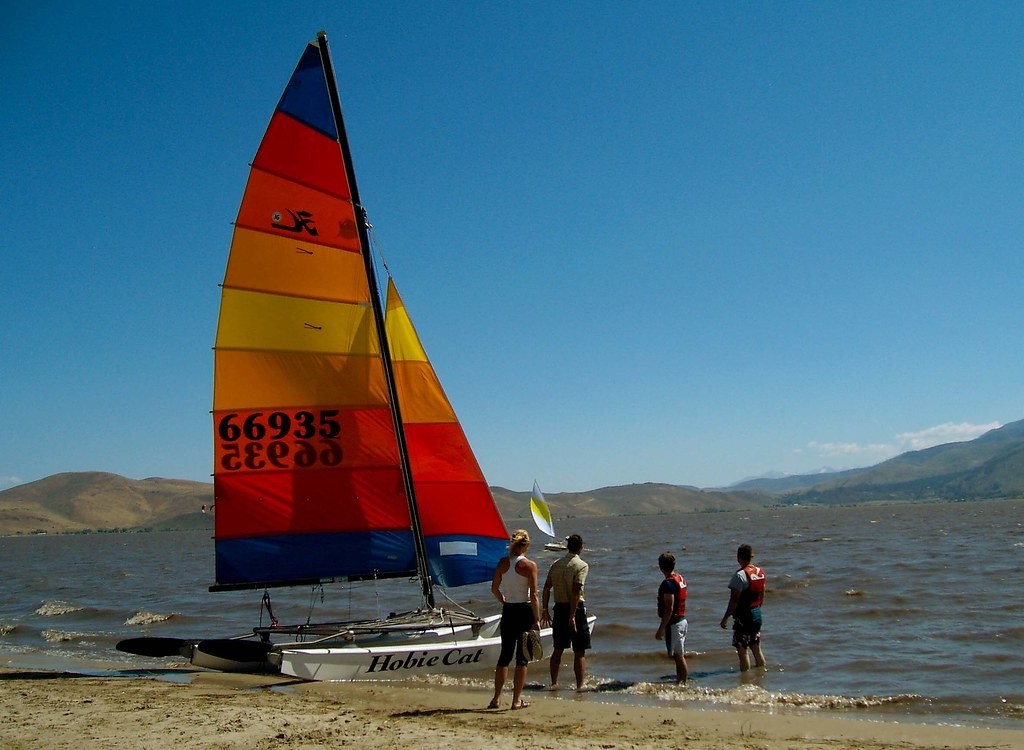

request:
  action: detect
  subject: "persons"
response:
[655,552,688,684]
[540,534,592,689]
[487,529,540,710]
[720,544,766,670]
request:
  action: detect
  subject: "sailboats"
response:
[208,31,597,680]
[530,480,570,550]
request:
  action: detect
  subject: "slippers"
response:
[511,699,531,710]
[488,697,500,709]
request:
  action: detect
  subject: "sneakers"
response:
[529,630,544,661]
[521,632,533,662]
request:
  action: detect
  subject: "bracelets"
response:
[542,608,547,610]
